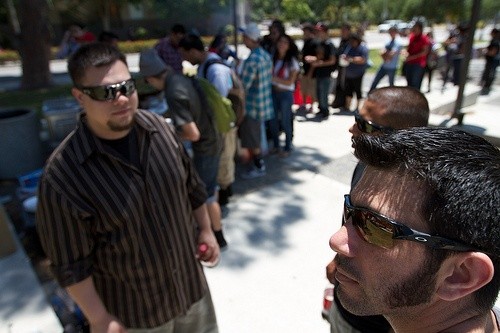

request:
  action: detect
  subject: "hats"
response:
[238,22,263,41]
[312,23,329,32]
[139,47,167,76]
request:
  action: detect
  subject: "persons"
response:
[326,86,431,333]
[368,17,500,94]
[329,127,500,333]
[37,43,220,333]
[155,19,300,73]
[57,18,99,61]
[177,35,238,206]
[235,23,274,180]
[268,35,300,158]
[296,20,370,120]
[139,48,229,250]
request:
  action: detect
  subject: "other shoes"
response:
[284,144,293,151]
[315,111,329,118]
[271,147,279,153]
[215,230,227,248]
[297,106,312,113]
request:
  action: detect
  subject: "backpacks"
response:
[202,59,246,126]
[190,75,237,133]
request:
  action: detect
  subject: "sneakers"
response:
[242,166,267,178]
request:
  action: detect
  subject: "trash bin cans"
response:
[0,108,38,179]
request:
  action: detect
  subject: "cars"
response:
[380,20,405,33]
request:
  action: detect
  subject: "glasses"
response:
[81,79,136,101]
[353,109,393,133]
[343,194,479,252]
